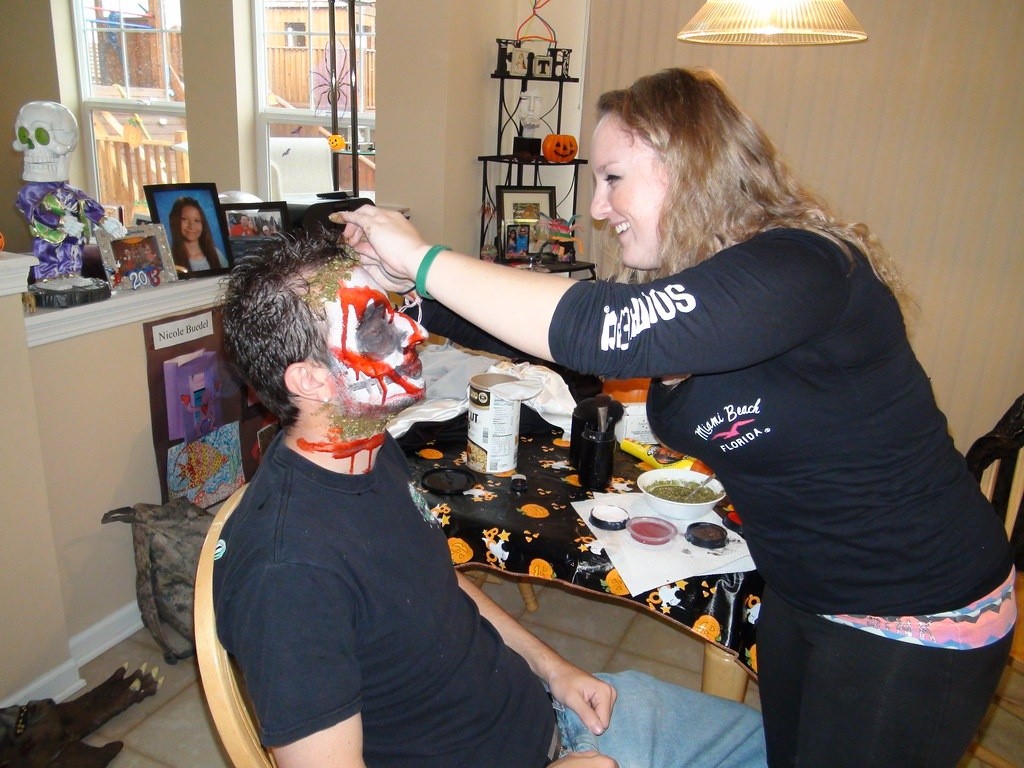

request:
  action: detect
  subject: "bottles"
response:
[466,373,521,474]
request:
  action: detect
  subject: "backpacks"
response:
[101,496,216,665]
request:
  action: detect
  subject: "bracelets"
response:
[415,244,454,299]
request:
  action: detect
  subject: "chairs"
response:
[189,478,284,768]
[957,389,1024,768]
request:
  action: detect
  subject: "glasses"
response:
[520,231,525,232]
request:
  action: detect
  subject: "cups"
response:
[578,422,616,490]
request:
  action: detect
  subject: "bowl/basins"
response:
[625,516,678,545]
[637,469,725,520]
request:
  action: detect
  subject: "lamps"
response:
[675,0,869,49]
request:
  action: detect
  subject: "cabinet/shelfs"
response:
[471,67,603,286]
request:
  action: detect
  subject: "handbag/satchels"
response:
[396,403,564,456]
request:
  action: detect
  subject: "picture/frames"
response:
[496,185,556,263]
[93,222,180,295]
[505,224,531,261]
[220,201,292,248]
[142,181,235,281]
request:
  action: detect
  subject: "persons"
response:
[13,101,127,292]
[516,227,528,253]
[508,229,517,253]
[328,68,1017,768]
[220,221,766,768]
[169,197,228,272]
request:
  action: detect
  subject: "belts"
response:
[545,691,562,768]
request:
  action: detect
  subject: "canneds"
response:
[466,373,544,474]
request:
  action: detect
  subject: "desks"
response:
[385,349,766,708]
